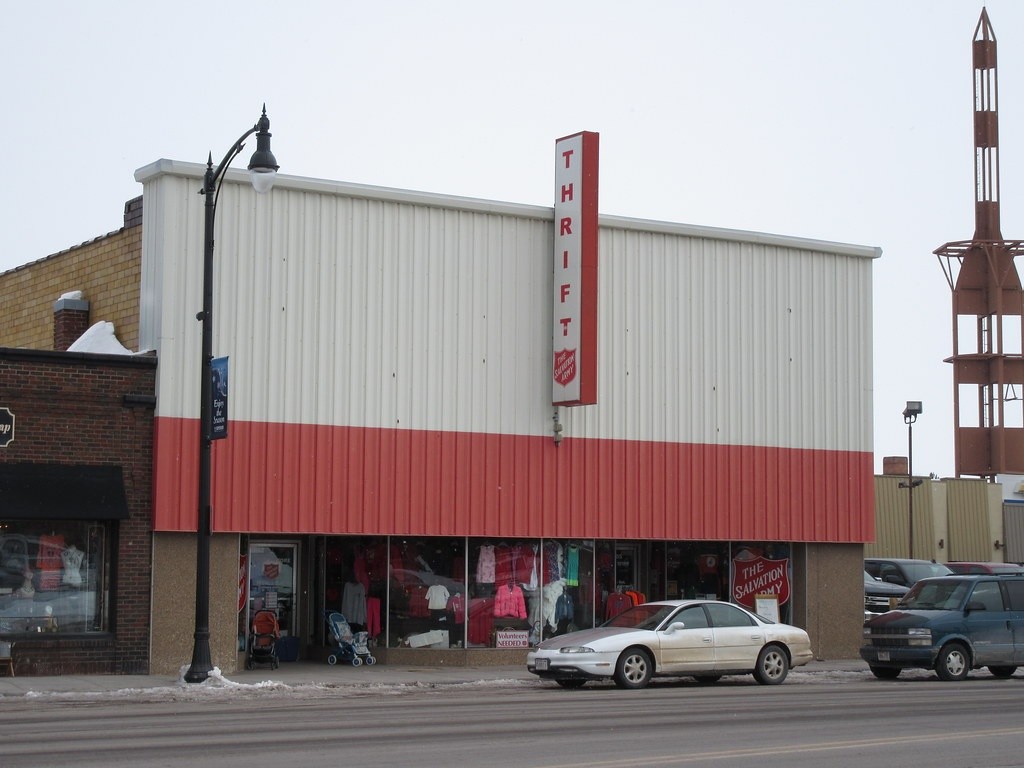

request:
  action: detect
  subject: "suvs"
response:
[865,569,916,625]
[864,557,958,603]
[941,560,1024,594]
[858,570,1024,683]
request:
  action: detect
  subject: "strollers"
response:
[246,607,280,671]
[328,613,377,667]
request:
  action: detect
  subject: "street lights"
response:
[189,100,282,685]
[899,400,924,558]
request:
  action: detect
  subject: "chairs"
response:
[0,641,15,677]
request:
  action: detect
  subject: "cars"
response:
[393,567,464,620]
[529,599,814,690]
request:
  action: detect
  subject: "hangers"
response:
[497,539,512,550]
[475,540,500,550]
[514,538,526,549]
